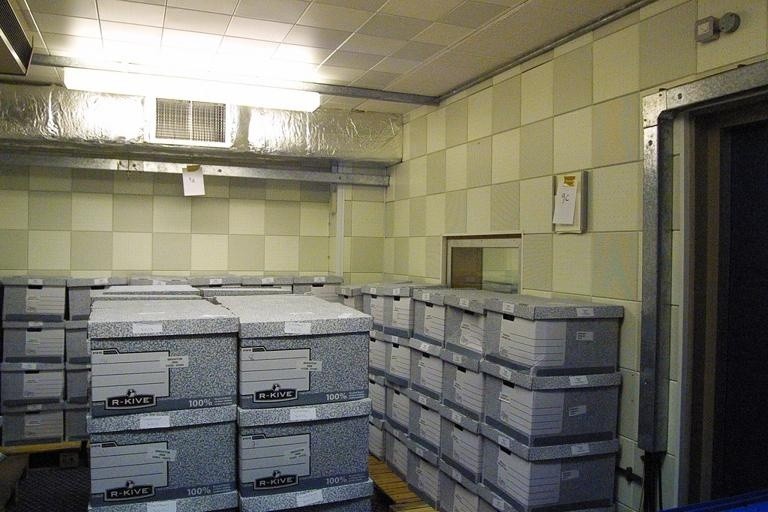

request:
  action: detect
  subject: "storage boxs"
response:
[2,273,132,447]
[88,274,373,510]
[361,273,626,510]
